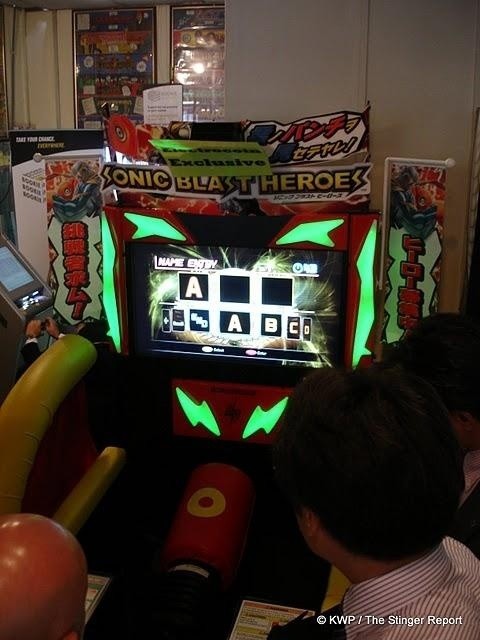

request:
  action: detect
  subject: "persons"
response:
[14,314,108,378]
[396,310,480,560]
[0,509,89,639]
[263,360,480,640]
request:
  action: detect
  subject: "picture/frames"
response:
[72,3,225,130]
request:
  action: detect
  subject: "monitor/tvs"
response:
[125,242,347,371]
[0,245,36,293]
